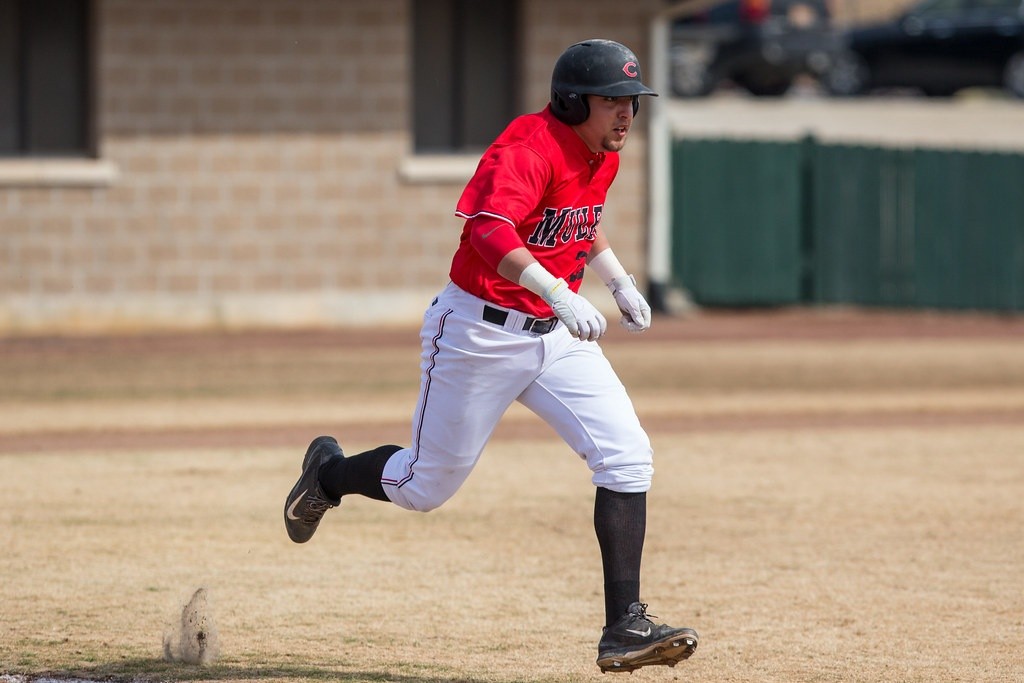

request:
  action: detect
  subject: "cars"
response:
[668,0,833,99]
[821,0,1024,102]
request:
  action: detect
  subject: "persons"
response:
[282,37,700,672]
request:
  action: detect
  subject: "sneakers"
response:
[597,601,698,674]
[284,436,345,543]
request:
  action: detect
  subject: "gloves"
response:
[605,274,652,334]
[544,278,607,342]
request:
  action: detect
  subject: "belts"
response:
[483,305,559,337]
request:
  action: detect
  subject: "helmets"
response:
[551,39,658,126]
